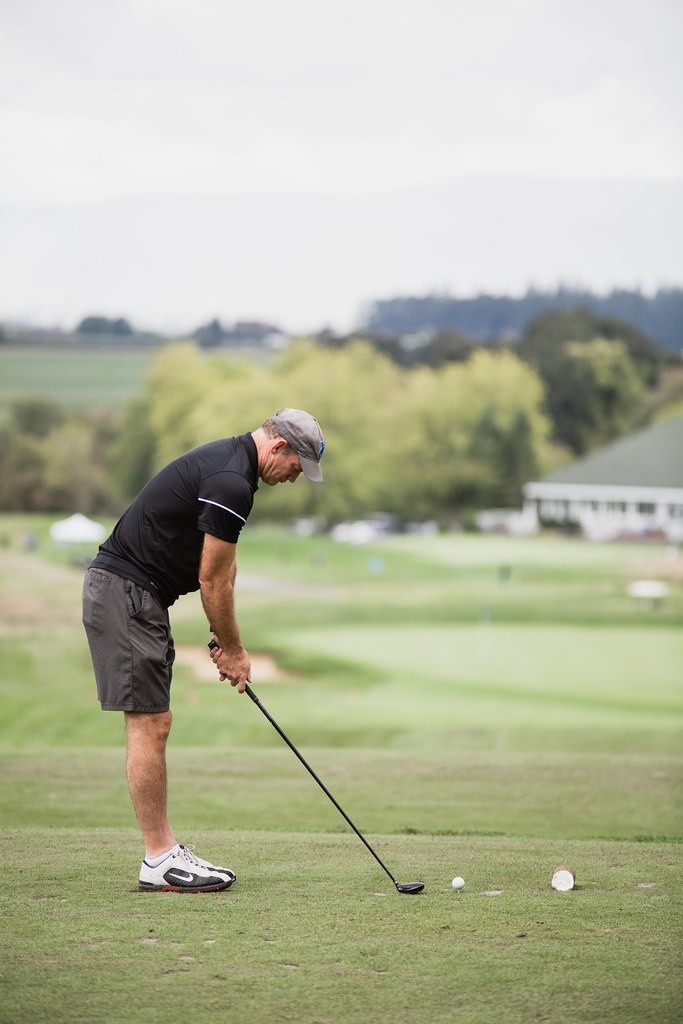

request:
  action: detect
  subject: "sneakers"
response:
[138,844,236,892]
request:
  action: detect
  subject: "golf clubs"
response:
[206,639,428,894]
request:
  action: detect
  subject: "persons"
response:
[81,409,325,893]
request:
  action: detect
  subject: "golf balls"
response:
[452,877,464,890]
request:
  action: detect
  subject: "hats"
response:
[272,408,325,483]
